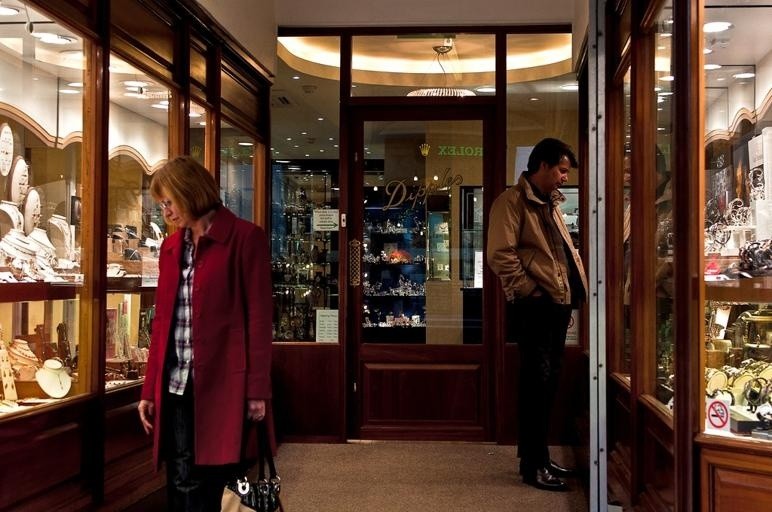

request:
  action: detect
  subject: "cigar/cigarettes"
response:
[711,414,724,417]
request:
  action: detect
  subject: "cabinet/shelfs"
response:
[1,0,270,511]
[273,169,427,346]
[568,6,769,512]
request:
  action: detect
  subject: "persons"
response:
[623,143,672,328]
[486,138,589,492]
[136,156,276,512]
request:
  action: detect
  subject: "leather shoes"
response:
[519,461,576,492]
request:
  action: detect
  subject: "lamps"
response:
[407,46,476,97]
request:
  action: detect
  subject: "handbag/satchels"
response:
[220,478,284,512]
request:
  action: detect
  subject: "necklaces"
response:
[2,205,72,256]
[8,342,65,391]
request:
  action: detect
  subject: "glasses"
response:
[158,200,173,209]
